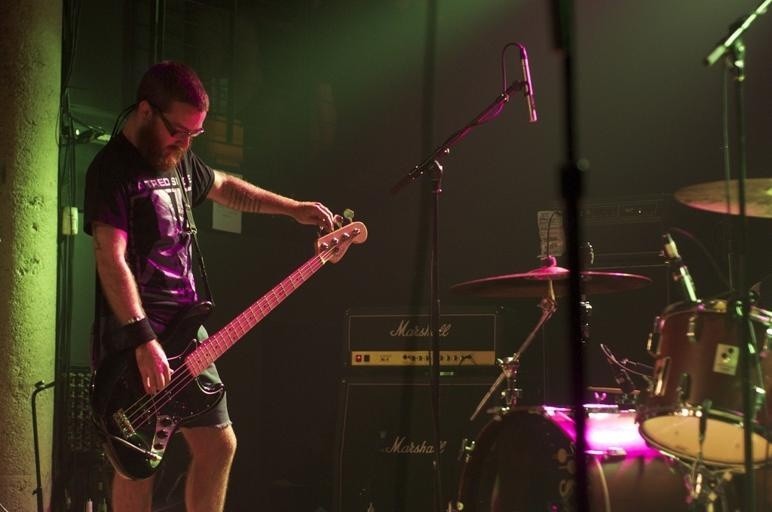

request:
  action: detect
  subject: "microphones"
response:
[662,233,698,307]
[521,47,539,125]
[705,1,771,66]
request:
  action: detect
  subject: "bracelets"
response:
[118,316,158,352]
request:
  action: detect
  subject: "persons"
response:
[83,61,338,512]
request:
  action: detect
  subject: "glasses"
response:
[152,106,205,140]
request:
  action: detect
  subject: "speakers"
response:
[329,303,515,511]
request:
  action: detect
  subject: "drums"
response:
[445,403,731,512]
[635,299,772,467]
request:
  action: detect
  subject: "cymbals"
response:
[449,263,650,302]
[672,177,772,218]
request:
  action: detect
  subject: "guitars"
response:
[88,208,369,483]
